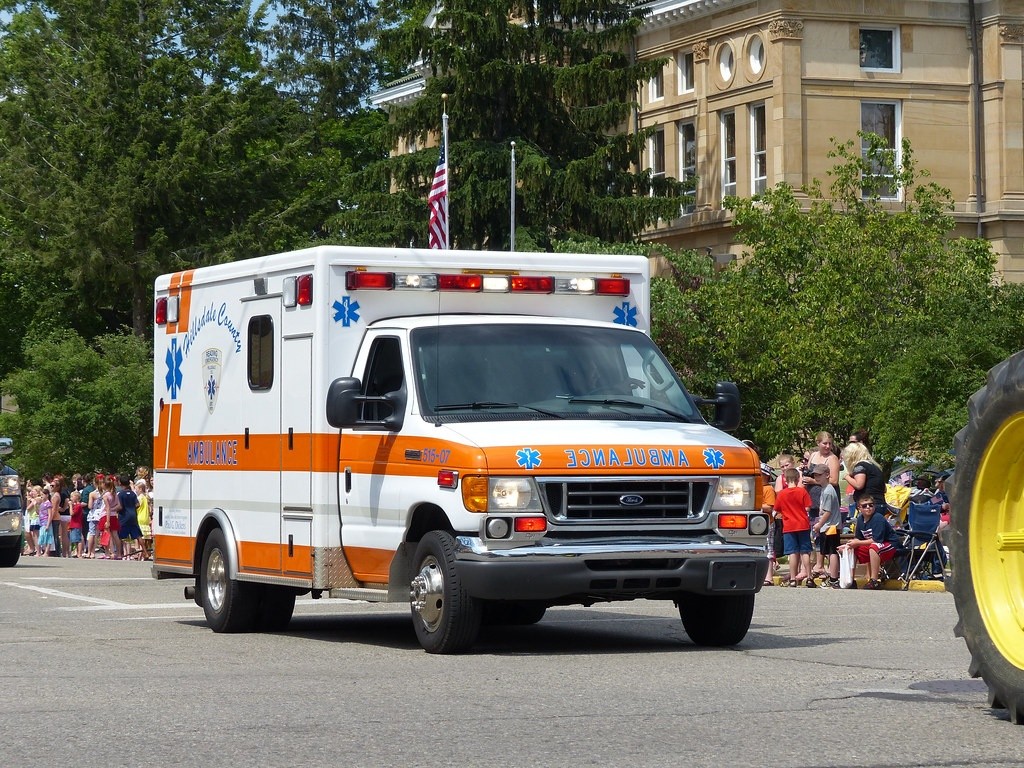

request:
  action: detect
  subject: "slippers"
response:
[762,580,774,586]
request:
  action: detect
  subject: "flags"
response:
[426,121,448,249]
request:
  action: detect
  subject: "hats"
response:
[812,464,830,474]
[760,463,771,475]
[849,436,857,441]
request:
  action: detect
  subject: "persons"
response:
[24,466,154,561]
[742,431,953,589]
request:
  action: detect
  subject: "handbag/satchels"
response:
[773,511,784,558]
[910,501,942,534]
[100,525,110,547]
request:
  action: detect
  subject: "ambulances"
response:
[149,245,775,655]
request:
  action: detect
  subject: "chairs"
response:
[880,502,946,591]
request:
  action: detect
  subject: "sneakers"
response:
[805,578,817,588]
[863,579,881,590]
[832,581,857,589]
[820,580,834,589]
[790,580,797,587]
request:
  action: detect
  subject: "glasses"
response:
[860,503,873,509]
[50,485,56,487]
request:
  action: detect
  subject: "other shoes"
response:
[21,550,151,562]
